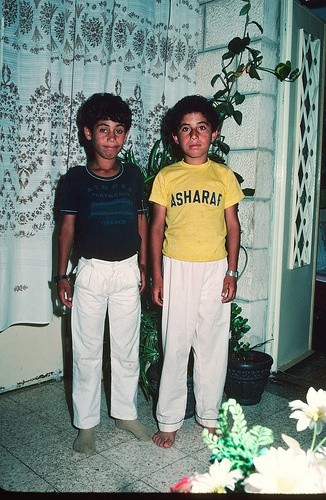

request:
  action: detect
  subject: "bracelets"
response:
[54,275,67,281]
[139,264,145,272]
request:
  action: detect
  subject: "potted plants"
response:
[139,310,195,420]
[224,302,274,407]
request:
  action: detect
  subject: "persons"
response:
[148,95,245,448]
[54,93,155,453]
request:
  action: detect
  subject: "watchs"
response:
[227,270,238,278]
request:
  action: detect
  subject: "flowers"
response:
[170,386,326,494]
